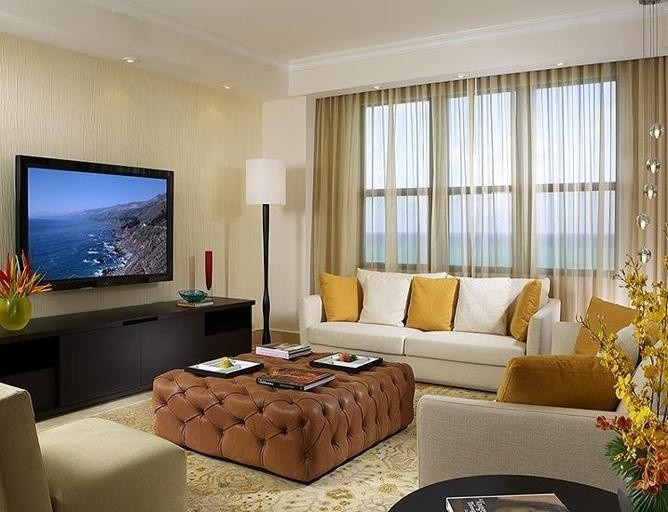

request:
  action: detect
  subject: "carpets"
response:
[86,382,497,512]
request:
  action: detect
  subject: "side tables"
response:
[387,474,621,511]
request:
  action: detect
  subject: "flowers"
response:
[573,222,668,512]
[0,249,52,319]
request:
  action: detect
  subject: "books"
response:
[446,493,569,512]
[254,341,312,360]
[257,368,336,391]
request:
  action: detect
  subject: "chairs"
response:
[0,382,188,512]
[416,321,668,512]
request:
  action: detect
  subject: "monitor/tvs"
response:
[15,155,173,291]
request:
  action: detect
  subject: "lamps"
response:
[245,158,286,345]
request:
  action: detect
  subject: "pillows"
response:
[495,353,620,412]
[573,296,643,355]
[319,272,552,343]
[508,277,551,322]
[357,267,447,287]
[596,325,640,368]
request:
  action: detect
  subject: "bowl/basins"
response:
[179,289,207,303]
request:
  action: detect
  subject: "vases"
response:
[0,298,33,331]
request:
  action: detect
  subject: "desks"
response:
[0,296,256,423]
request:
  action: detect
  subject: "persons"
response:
[448,497,568,512]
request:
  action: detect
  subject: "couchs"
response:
[298,267,561,393]
